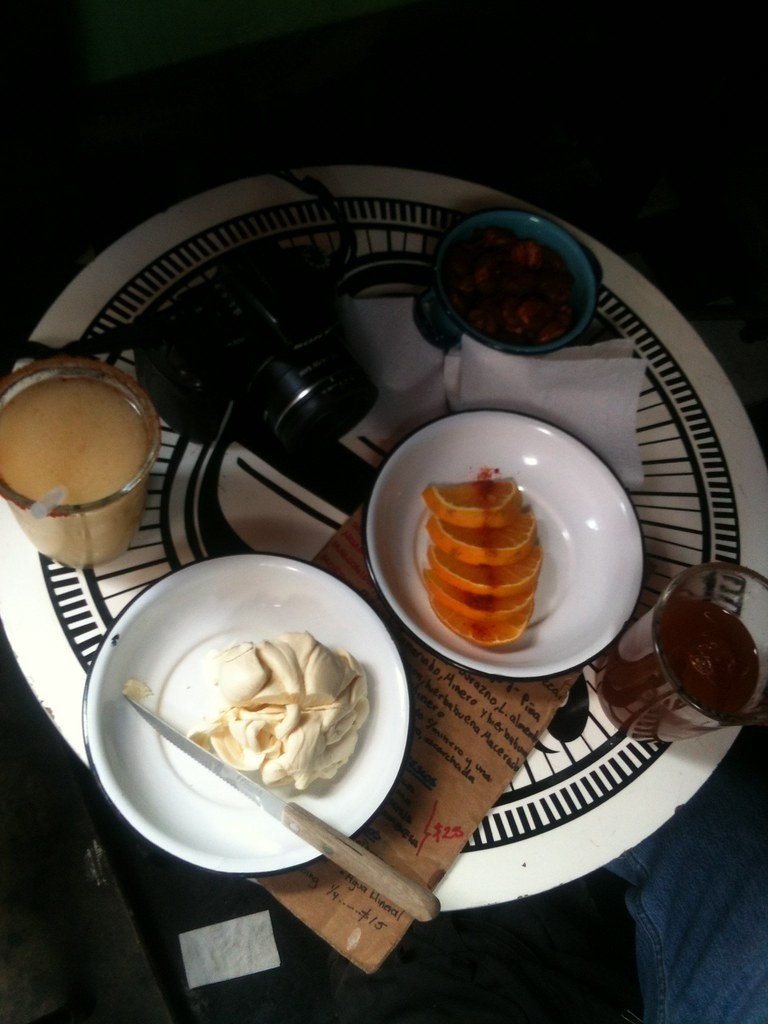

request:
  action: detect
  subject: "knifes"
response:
[123,693,441,923]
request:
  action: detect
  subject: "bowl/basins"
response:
[432,207,598,356]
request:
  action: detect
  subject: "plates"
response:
[82,551,414,878]
[360,406,648,684]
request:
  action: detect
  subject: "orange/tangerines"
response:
[420,476,543,646]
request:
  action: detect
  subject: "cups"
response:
[0,357,161,570]
[594,562,768,744]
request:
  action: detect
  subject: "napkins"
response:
[337,296,645,488]
